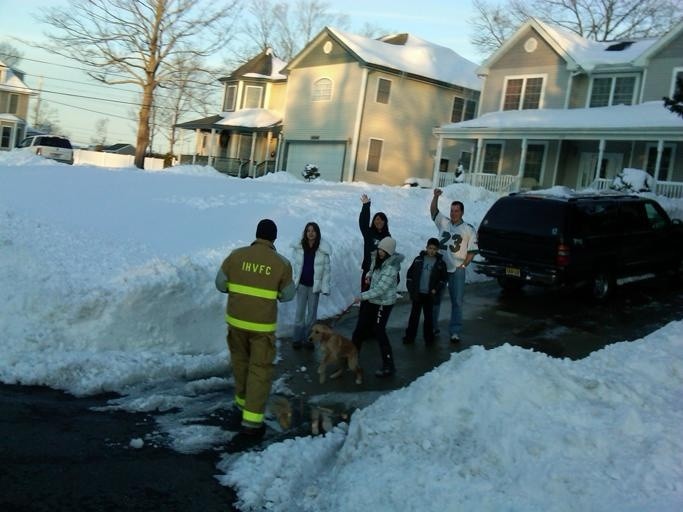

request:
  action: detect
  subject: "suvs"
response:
[16,134,74,166]
[467,185,682,302]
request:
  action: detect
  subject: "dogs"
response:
[308,324,362,384]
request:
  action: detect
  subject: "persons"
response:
[401,238,448,346]
[264,395,294,433]
[215,218,296,437]
[356,192,391,312]
[429,188,479,342]
[289,223,332,349]
[354,237,405,377]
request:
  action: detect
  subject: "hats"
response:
[378,236,396,256]
[256,218,277,242]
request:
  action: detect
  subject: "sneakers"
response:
[375,366,397,378]
[303,340,315,352]
[402,331,417,344]
[422,329,441,346]
[291,340,304,350]
[240,421,266,437]
[449,332,460,343]
